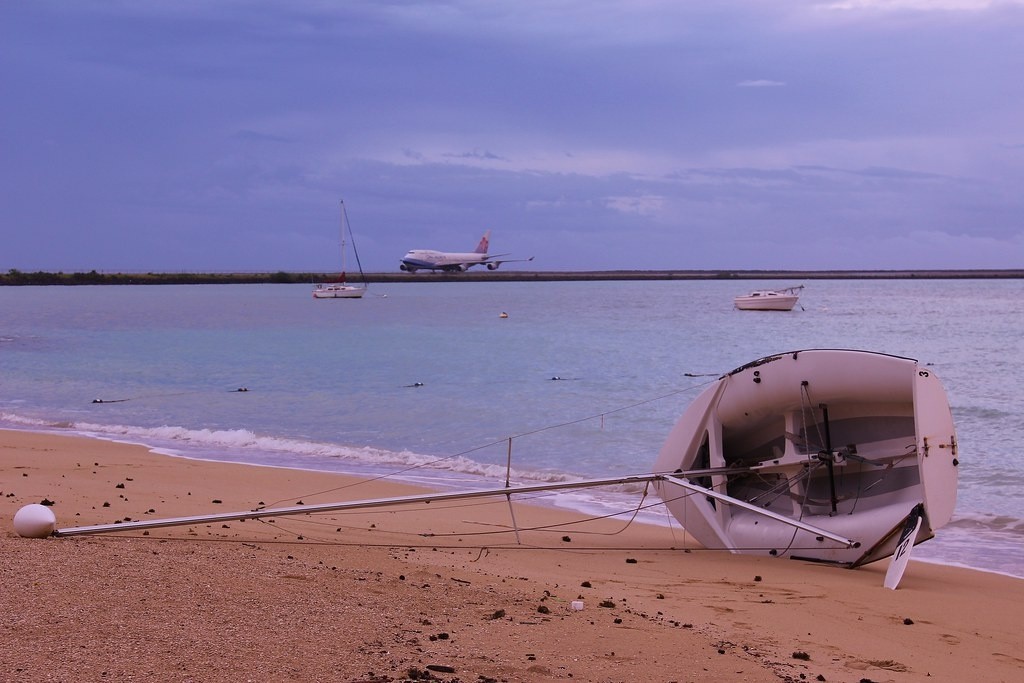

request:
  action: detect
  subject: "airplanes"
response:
[399,227,535,275]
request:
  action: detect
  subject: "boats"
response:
[733,281,805,313]
[15,346,959,585]
[311,199,370,298]
[499,311,508,318]
